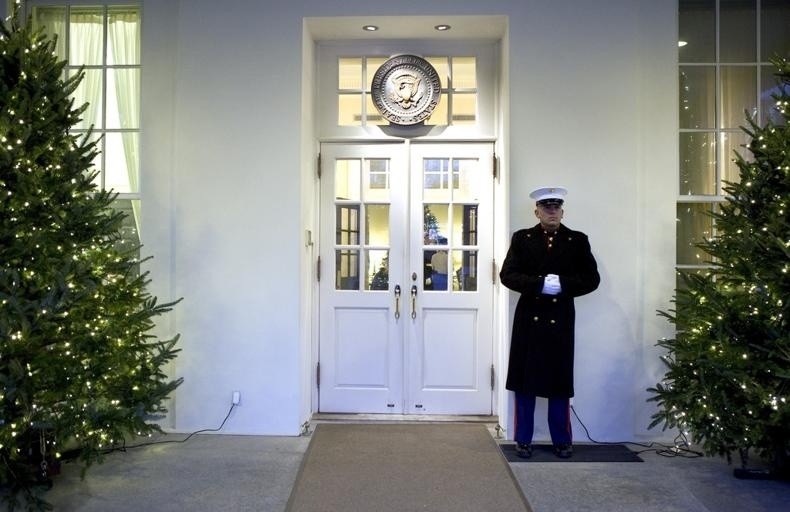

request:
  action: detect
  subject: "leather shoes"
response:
[515,441,533,459]
[553,442,573,458]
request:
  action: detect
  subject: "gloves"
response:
[540,273,562,296]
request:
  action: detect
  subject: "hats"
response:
[527,184,569,207]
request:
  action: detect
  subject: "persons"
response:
[370,250,389,290]
[424,232,447,290]
[500,187,601,458]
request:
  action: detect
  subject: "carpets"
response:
[285,420,531,511]
[499,444,645,463]
